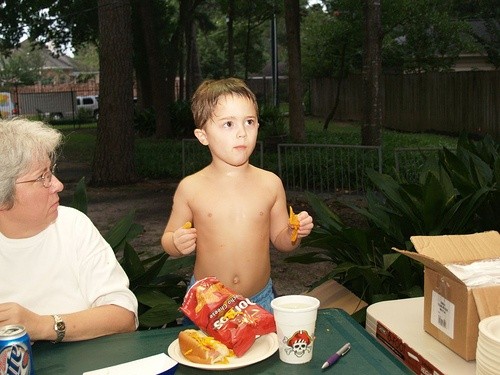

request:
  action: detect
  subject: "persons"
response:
[160,77,315,317]
[0,118,139,343]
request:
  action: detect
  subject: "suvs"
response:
[75,96,100,120]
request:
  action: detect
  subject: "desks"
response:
[30,308,416,375]
[366,297,476,375]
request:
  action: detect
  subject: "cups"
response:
[270,295,320,365]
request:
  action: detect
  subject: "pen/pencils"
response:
[321,341,351,369]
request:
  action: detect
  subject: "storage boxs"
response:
[391,231,500,362]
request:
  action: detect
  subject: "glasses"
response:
[15,163,57,188]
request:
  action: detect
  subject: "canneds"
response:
[0,325,35,375]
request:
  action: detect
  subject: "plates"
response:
[167,332,279,371]
[475,315,500,375]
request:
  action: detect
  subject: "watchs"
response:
[48,313,66,344]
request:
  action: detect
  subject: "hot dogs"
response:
[179,329,230,365]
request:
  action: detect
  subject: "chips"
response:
[194,283,233,312]
